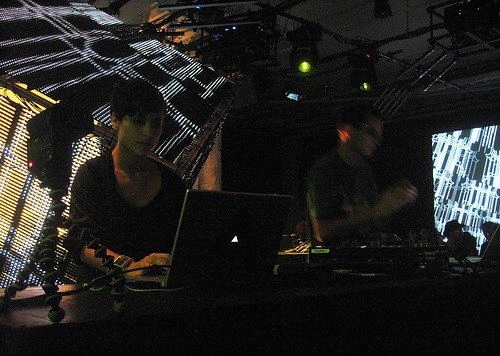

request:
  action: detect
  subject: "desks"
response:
[0,269,500,356]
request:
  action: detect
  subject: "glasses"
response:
[355,121,384,143]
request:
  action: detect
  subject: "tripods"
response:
[0,175,126,323]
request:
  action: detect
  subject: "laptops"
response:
[450,225,500,266]
[126,189,293,288]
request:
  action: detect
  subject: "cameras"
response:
[26,102,94,181]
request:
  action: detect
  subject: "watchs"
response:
[114,254,135,269]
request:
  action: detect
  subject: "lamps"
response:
[286,26,322,76]
[373,0,393,19]
[351,65,379,93]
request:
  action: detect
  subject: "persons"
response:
[67,80,185,280]
[294,104,500,262]
